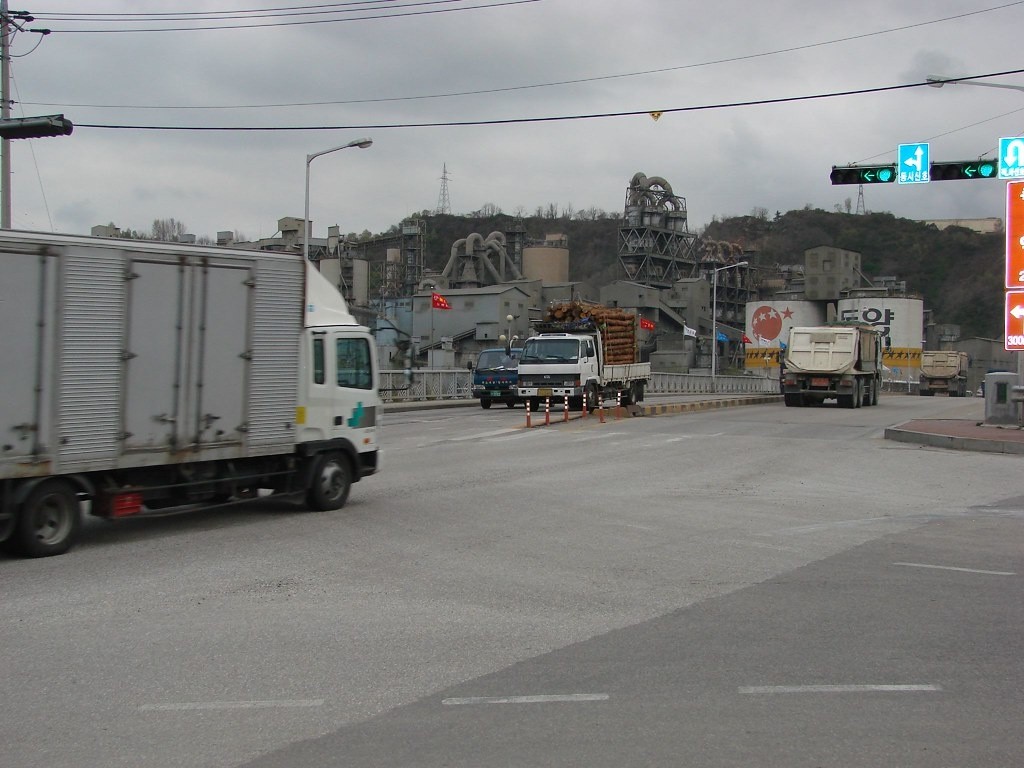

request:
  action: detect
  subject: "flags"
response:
[741,333,752,343]
[432,292,453,310]
[684,325,696,337]
[717,329,728,342]
[780,342,786,348]
[758,337,771,347]
[882,366,903,375]
[640,317,655,332]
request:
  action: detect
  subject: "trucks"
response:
[516,318,653,415]
[0,227,415,559]
[467,347,525,410]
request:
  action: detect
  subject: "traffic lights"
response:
[930,159,997,181]
[829,163,899,185]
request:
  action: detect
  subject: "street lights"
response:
[711,262,749,381]
[304,137,377,263]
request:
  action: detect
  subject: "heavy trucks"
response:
[782,321,892,409]
[919,350,972,397]
